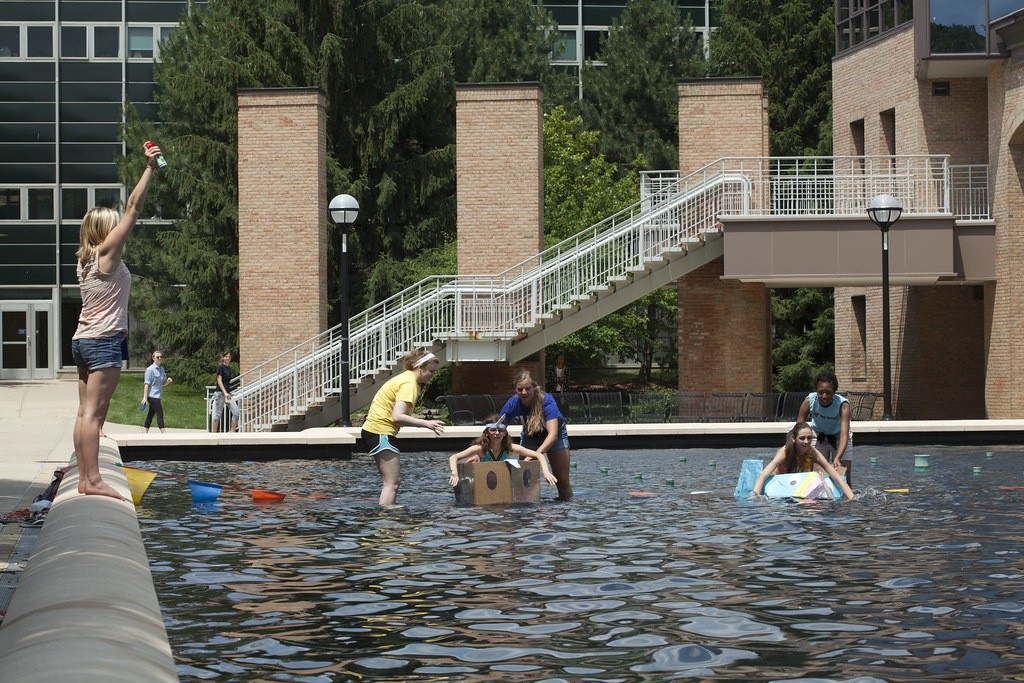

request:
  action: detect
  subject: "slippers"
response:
[20,513,46,528]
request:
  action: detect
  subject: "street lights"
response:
[864,195,902,420]
[328,194,361,426]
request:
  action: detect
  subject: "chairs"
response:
[743,391,785,422]
[846,391,866,421]
[584,392,629,423]
[836,392,848,398]
[707,392,747,422]
[851,392,886,420]
[626,394,666,422]
[436,391,585,426]
[777,391,810,421]
[668,391,710,423]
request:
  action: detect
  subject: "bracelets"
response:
[146,167,155,169]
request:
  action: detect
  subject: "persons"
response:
[72,141,163,501]
[796,372,853,489]
[361,350,446,505]
[500,370,573,496]
[545,353,571,418]
[753,421,854,500]
[141,350,172,433]
[212,349,240,433]
[448,414,557,488]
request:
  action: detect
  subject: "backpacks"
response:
[32,471,63,504]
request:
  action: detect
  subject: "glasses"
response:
[154,356,162,359]
